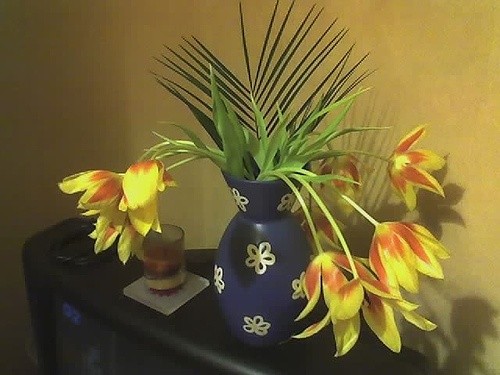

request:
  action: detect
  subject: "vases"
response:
[212,163,313,347]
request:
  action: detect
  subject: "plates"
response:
[122,271,210,316]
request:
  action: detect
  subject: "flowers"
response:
[57,0,449,359]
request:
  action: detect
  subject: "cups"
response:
[142,223,185,297]
